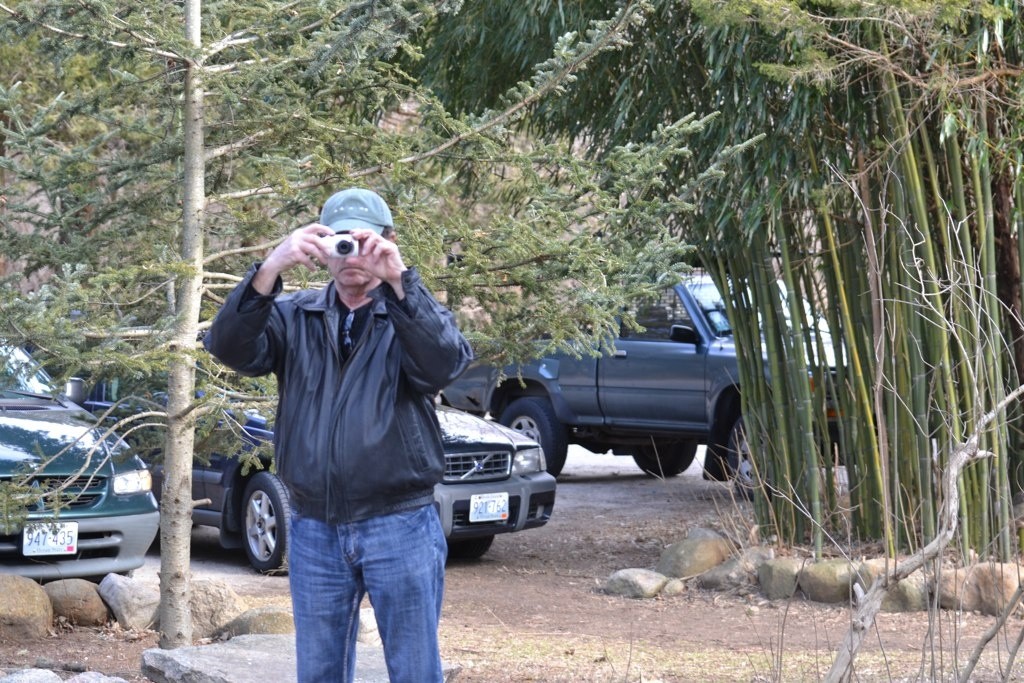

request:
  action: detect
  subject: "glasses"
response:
[343,311,355,357]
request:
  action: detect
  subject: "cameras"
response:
[320,233,359,258]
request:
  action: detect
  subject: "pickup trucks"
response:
[0,336,160,577]
[81,380,556,576]
[441,273,849,500]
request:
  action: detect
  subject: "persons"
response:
[203,188,473,683]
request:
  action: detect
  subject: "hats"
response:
[320,188,394,235]
